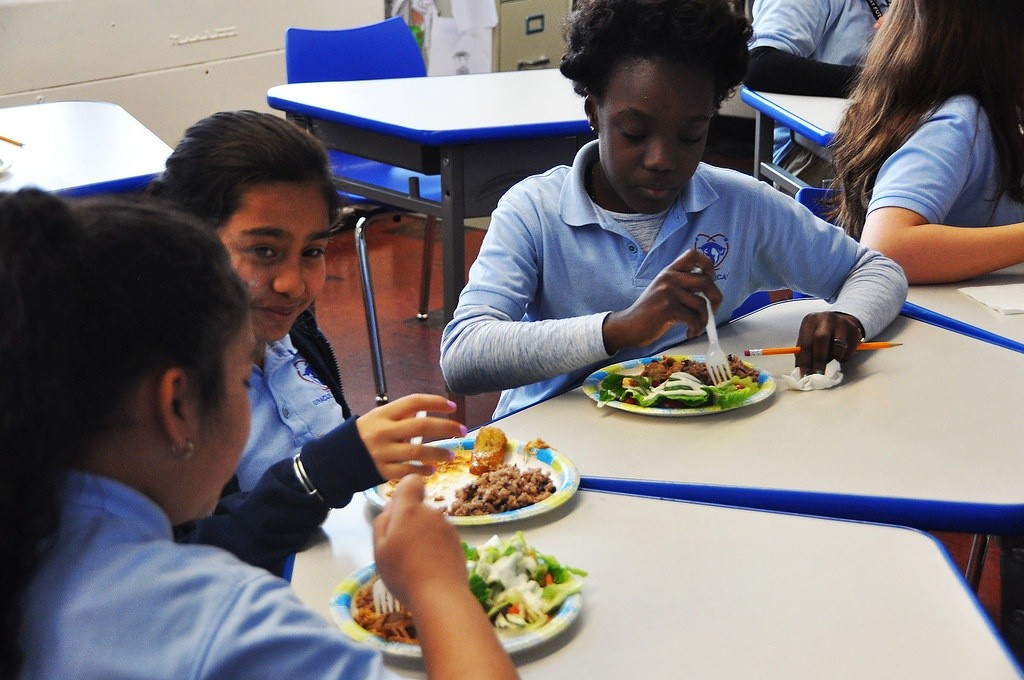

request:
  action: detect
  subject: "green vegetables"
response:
[463,529,588,633]
[597,372,760,411]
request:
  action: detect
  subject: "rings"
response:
[833,338,848,349]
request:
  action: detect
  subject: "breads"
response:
[469,427,507,474]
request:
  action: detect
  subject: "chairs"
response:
[285,15,450,406]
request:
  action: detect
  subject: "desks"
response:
[0,101,177,201]
[740,83,881,196]
[267,66,609,431]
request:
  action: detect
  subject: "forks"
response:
[373,578,400,617]
[691,266,733,386]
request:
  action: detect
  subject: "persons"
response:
[743,0,893,198]
[160,109,467,578]
[0,186,520,680]
[833,0,1024,284]
[440,0,908,422]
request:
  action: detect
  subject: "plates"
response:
[332,545,583,661]
[361,437,580,527]
[583,353,778,419]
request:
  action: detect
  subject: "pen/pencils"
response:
[744,341,903,356]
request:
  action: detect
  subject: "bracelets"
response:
[295,454,335,512]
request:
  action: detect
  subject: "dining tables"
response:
[270,256,1024,680]
